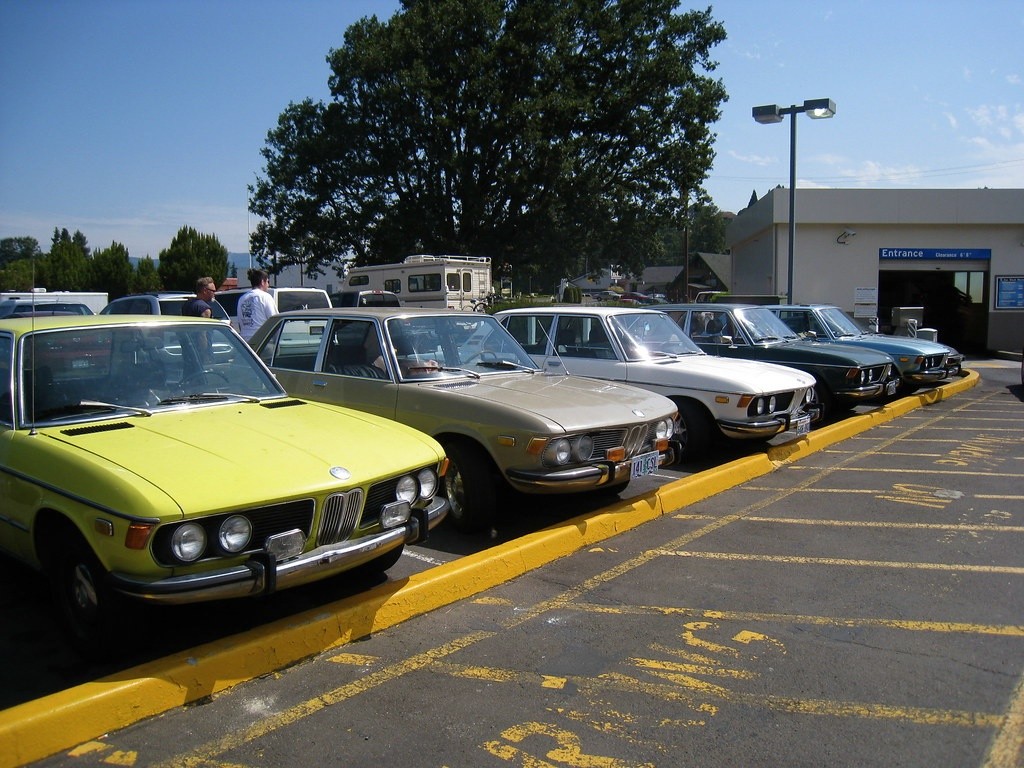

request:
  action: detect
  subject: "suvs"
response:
[0,298,93,316]
[695,291,721,304]
[622,292,648,302]
[97,292,234,367]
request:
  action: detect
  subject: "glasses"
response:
[206,288,216,293]
[267,281,269,284]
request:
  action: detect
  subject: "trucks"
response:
[339,254,492,313]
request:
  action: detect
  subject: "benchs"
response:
[264,353,320,372]
[53,373,108,401]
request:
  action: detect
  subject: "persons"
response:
[182,277,216,379]
[237,269,280,360]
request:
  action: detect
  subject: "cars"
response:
[0,314,455,641]
[0,310,112,383]
[647,298,669,305]
[435,305,822,456]
[593,291,622,301]
[230,307,678,532]
[640,303,901,414]
[761,306,963,398]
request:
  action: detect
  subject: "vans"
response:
[211,286,339,361]
[328,289,401,307]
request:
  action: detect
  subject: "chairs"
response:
[589,328,631,359]
[707,320,724,344]
[107,358,166,408]
[339,347,386,380]
[25,365,70,422]
[689,319,707,344]
[557,329,597,360]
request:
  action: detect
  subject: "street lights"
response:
[753,99,836,316]
